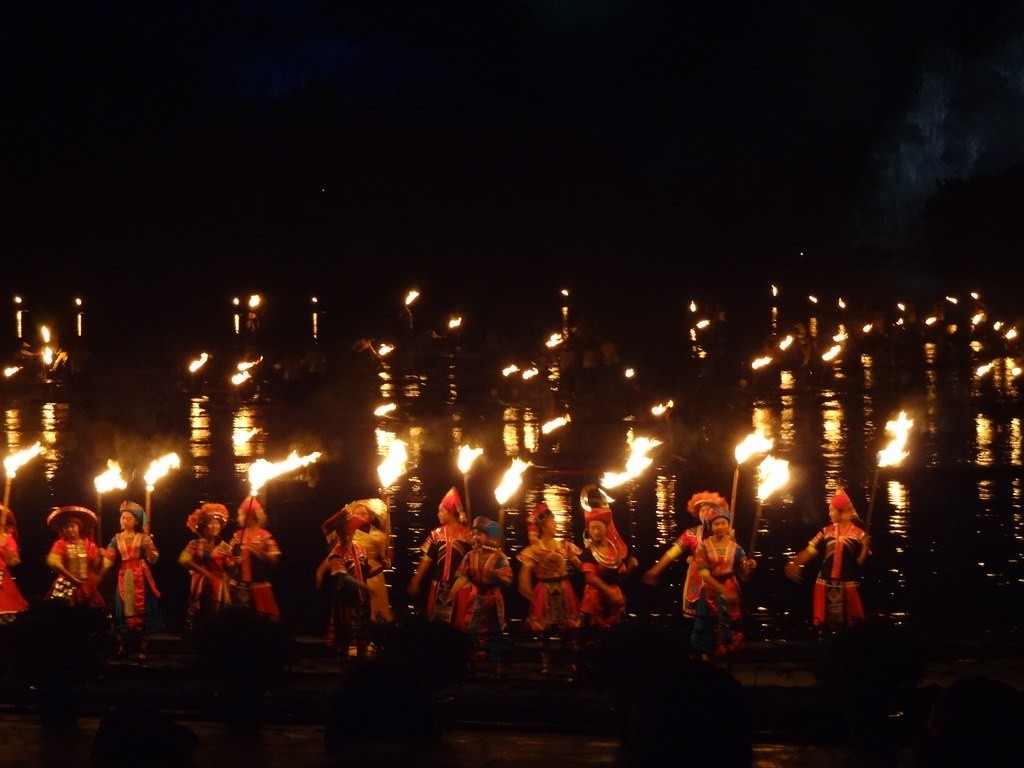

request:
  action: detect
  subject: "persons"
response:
[449,515,513,675]
[1,504,29,624]
[643,492,757,669]
[515,489,638,677]
[101,500,159,662]
[315,499,393,671]
[409,486,474,623]
[45,505,101,619]
[225,496,280,625]
[179,503,235,663]
[785,490,872,673]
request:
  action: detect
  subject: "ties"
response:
[323,508,371,544]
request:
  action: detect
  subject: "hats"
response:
[442,486,468,525]
[186,503,228,533]
[703,503,730,529]
[832,484,858,520]
[344,497,390,531]
[46,506,97,535]
[584,508,613,524]
[473,517,500,536]
[529,505,553,524]
[242,496,267,527]
[120,501,146,525]
[686,491,729,517]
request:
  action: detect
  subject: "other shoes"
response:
[107,655,129,665]
[495,668,504,681]
[542,663,551,674]
